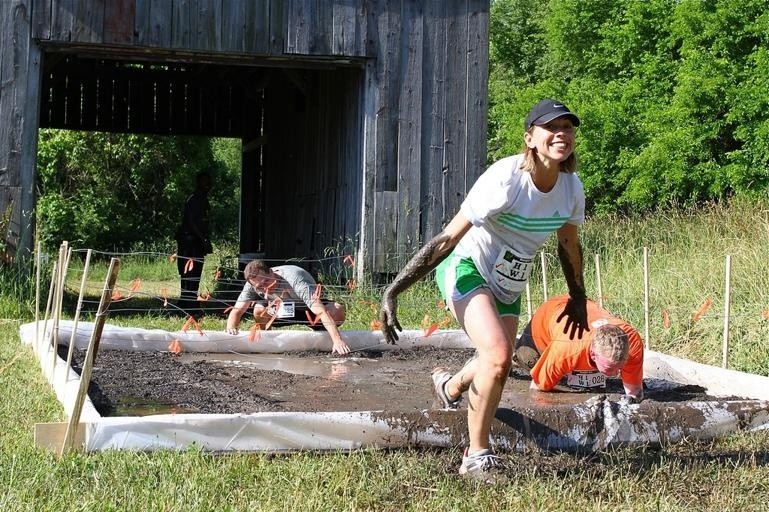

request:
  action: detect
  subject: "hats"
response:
[524,99,580,130]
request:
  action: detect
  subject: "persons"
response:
[513,297,644,401]
[224,259,350,357]
[175,171,215,304]
[380,99,590,486]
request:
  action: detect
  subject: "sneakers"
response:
[458,447,507,485]
[430,368,462,408]
